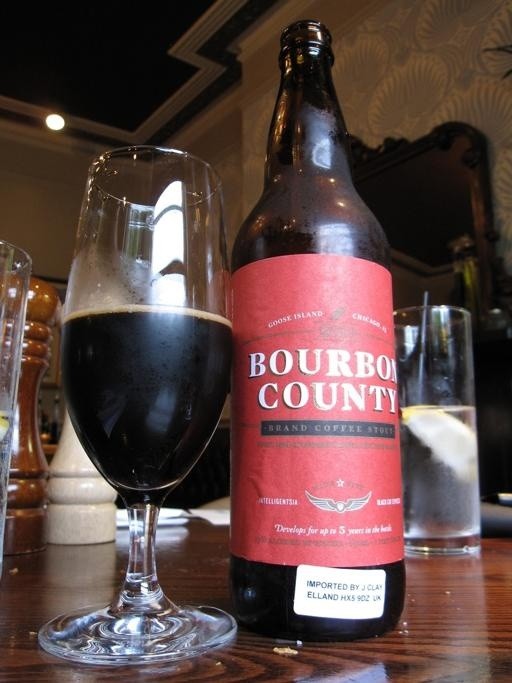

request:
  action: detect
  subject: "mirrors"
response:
[343,120,494,309]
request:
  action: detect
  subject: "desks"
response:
[0,506,512,683]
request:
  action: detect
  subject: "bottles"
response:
[445,229,512,541]
[229,15,408,649]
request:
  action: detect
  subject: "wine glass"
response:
[35,141,238,673]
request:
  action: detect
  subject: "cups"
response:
[0,240,34,591]
[394,304,483,562]
